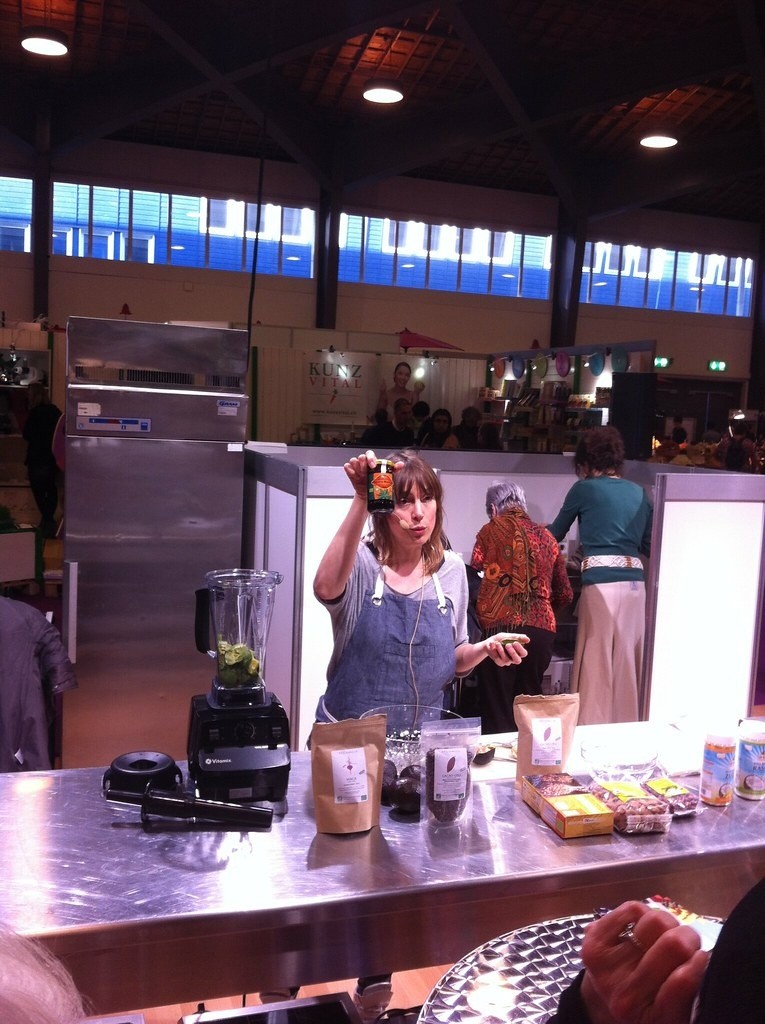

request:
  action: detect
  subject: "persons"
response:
[378,362,423,421]
[258,450,531,1024]
[541,426,654,726]
[658,416,759,472]
[535,878,765,1024]
[359,398,502,451]
[0,901,82,1024]
[471,482,573,735]
[22,383,63,539]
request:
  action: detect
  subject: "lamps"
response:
[488,354,512,371]
[529,352,556,371]
[581,346,612,368]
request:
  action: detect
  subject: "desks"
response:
[0,716,765,1016]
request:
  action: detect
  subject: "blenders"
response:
[187,567,292,814]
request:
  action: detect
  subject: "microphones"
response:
[390,512,410,531]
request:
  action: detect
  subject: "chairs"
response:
[0,596,79,773]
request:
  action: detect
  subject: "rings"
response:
[618,921,646,950]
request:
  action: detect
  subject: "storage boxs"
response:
[519,772,588,816]
[542,793,617,839]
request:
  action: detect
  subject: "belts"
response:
[580,555,644,570]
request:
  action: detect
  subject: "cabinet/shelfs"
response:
[493,396,519,419]
[535,399,568,431]
[565,406,609,438]
[511,406,542,438]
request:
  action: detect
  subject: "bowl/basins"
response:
[581,741,659,786]
[359,704,470,780]
